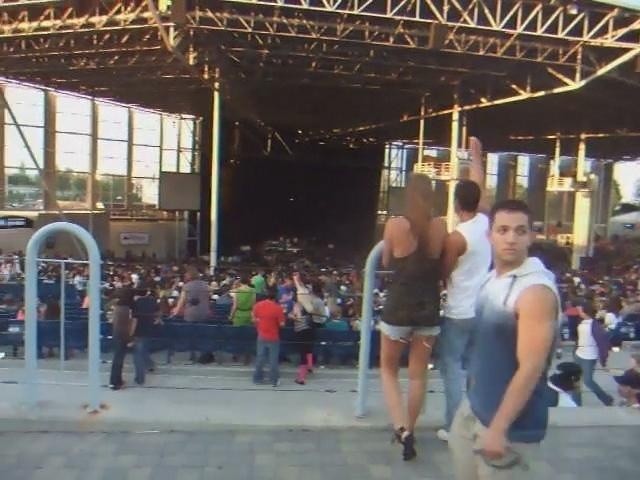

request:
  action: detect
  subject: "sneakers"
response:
[435,431,449,444]
[394,426,417,447]
[309,366,313,375]
[402,448,417,463]
[294,379,306,386]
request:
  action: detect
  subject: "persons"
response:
[562,257,640,301]
[436,178,492,441]
[228,273,256,362]
[109,277,136,390]
[221,269,238,289]
[320,306,350,331]
[170,265,211,362]
[101,260,169,281]
[287,270,313,385]
[216,283,233,305]
[570,302,614,407]
[128,281,157,384]
[352,298,362,330]
[447,199,562,479]
[146,281,162,312]
[332,268,362,297]
[254,266,270,296]
[545,362,584,408]
[0,248,89,284]
[612,368,640,407]
[251,287,287,387]
[263,269,276,300]
[379,173,447,460]
[0,291,60,356]
[321,296,345,323]
[373,288,381,304]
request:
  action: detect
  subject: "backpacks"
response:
[297,285,330,324]
